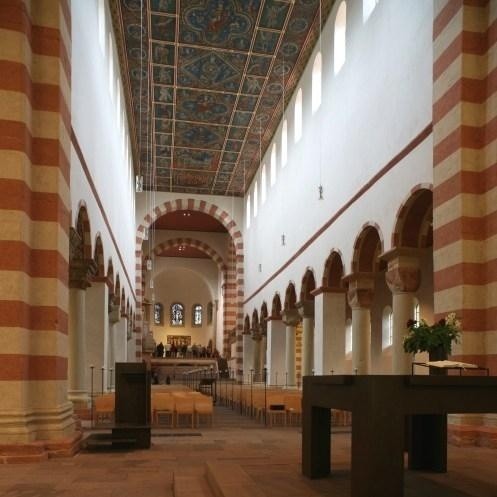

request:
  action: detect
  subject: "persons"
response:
[156,341,212,358]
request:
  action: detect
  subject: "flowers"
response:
[401,313,462,355]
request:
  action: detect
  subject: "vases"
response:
[429,352,449,377]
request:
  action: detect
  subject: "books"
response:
[426,360,478,368]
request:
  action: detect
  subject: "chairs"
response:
[94,379,352,428]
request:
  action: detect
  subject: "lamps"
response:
[258,113,261,273]
[281,57,286,247]
[135,1,158,304]
[314,2,325,201]
[233,158,248,293]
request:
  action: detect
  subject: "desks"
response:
[302,375,496,496]
